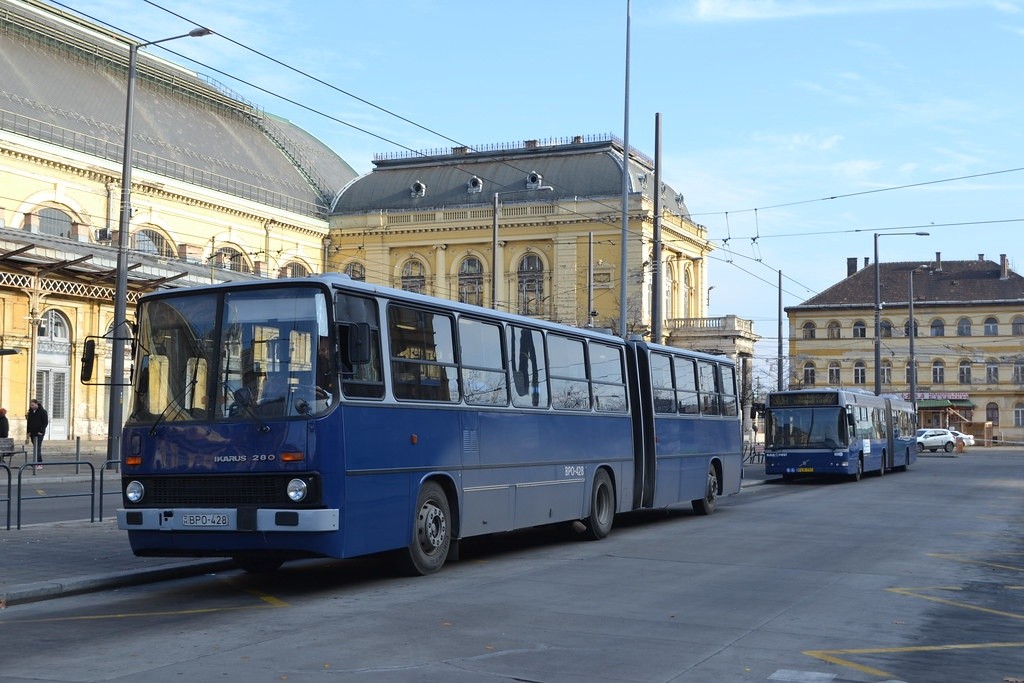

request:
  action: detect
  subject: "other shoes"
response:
[36,464,44,469]
[0,460,5,463]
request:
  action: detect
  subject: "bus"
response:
[750,386,919,482]
[80,273,744,577]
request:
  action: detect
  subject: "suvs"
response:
[914,428,957,453]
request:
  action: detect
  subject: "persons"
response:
[27,399,48,469]
[0,408,9,438]
[301,336,350,398]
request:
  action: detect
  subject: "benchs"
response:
[0,438,27,467]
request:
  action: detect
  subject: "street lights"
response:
[874,231,930,395]
[491,184,553,311]
[106,28,213,473]
[909,264,931,403]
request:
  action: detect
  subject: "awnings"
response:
[917,400,954,407]
[951,400,976,406]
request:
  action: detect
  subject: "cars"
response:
[949,431,975,447]
